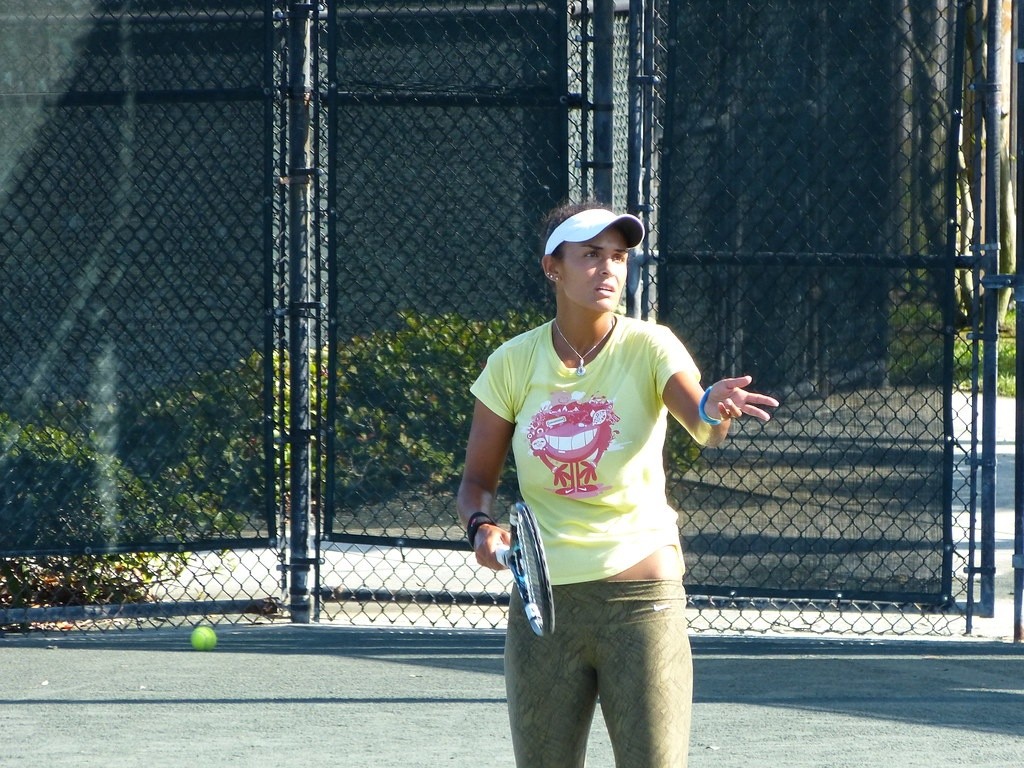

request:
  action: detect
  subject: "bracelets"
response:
[467,512,497,550]
[699,386,721,424]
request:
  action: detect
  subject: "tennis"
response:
[190,627,218,651]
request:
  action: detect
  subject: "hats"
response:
[544,209,646,256]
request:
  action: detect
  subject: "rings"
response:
[721,409,742,420]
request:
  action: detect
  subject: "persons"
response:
[457,203,779,768]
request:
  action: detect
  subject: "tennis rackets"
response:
[494,500,555,639]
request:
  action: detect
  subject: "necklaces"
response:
[555,319,614,376]
